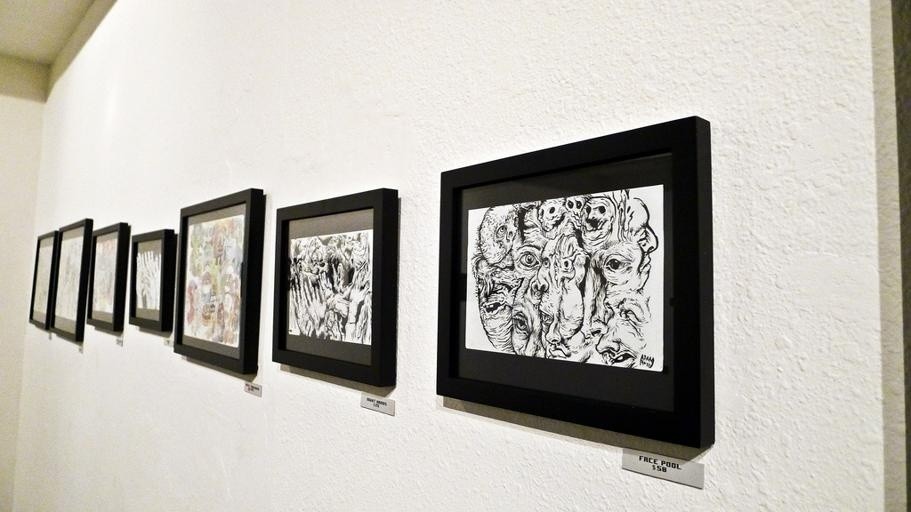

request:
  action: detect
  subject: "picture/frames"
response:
[437,116,715,449]
